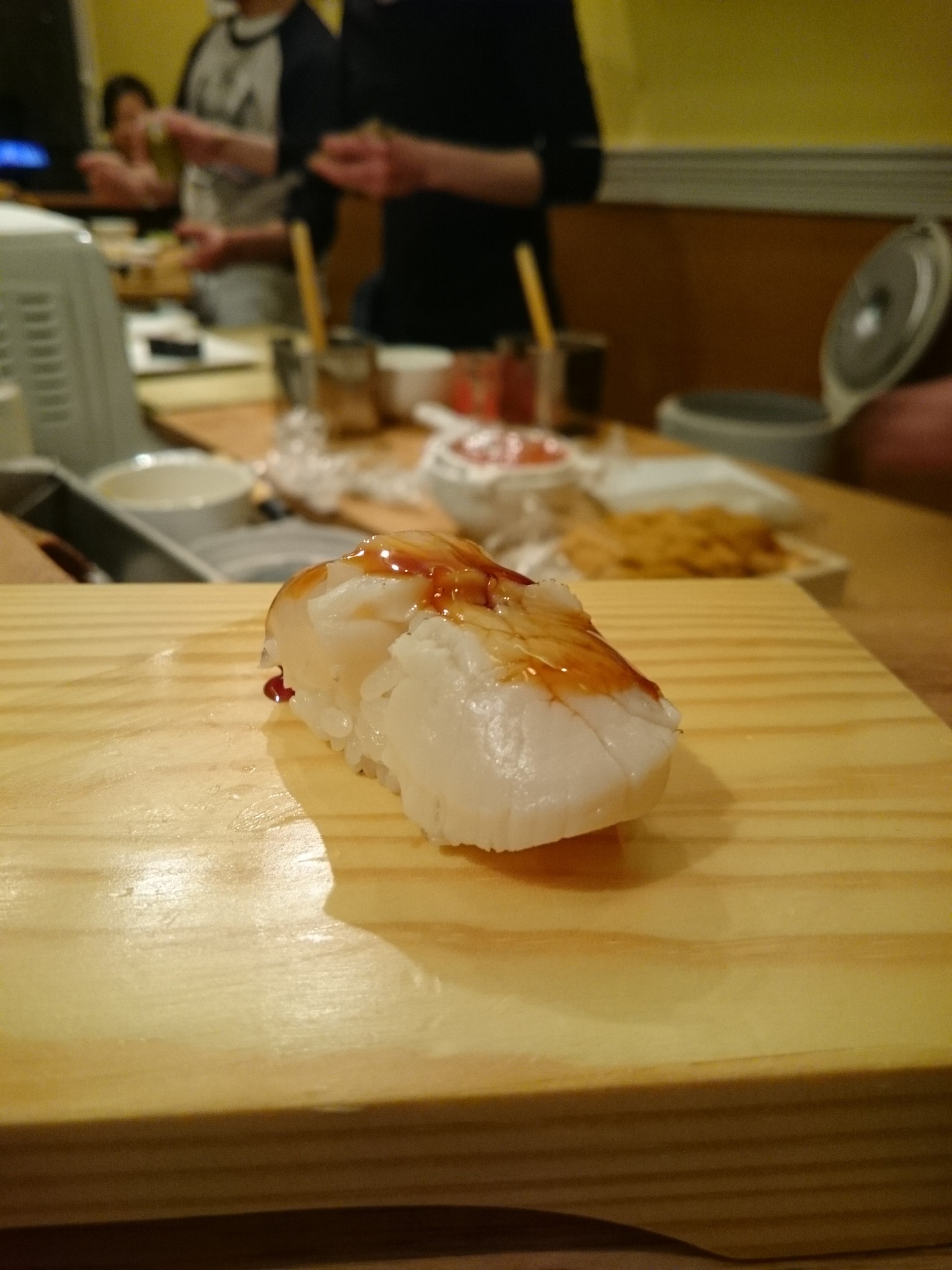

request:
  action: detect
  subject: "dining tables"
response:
[145,400,952,733]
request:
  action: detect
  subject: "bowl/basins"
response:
[88,453,256,550]
[418,436,584,539]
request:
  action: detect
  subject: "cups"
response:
[375,345,451,422]
[275,330,382,434]
[497,326,612,443]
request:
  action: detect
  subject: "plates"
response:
[198,520,379,585]
[515,520,848,616]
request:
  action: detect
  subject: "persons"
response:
[100,75,182,233]
[174,0,610,356]
[82,0,352,327]
